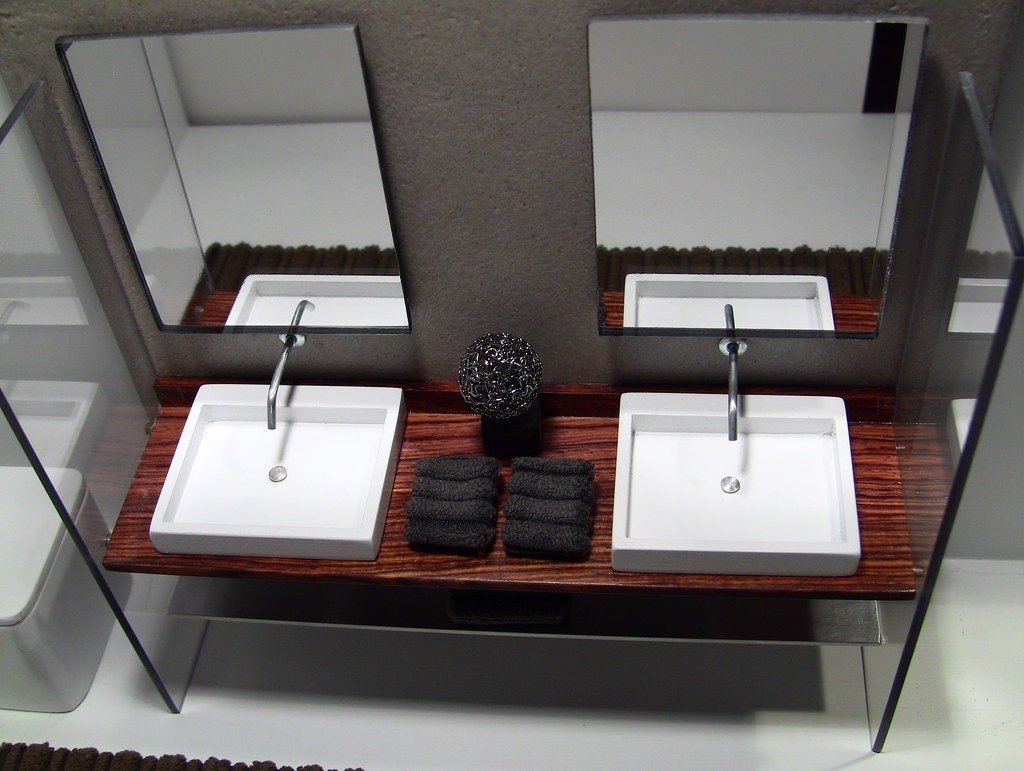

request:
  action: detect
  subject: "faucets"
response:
[266,336,293,432]
[724,303,737,330]
[728,345,737,443]
[290,300,317,325]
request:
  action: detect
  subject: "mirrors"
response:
[56,25,412,335]
[586,14,930,339]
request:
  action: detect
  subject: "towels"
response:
[402,453,503,558]
[503,456,603,561]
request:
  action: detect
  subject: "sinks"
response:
[621,273,835,332]
[945,396,978,473]
[1,379,109,472]
[2,276,161,327]
[612,393,861,576]
[224,274,408,326]
[146,383,406,561]
[944,279,1008,333]
